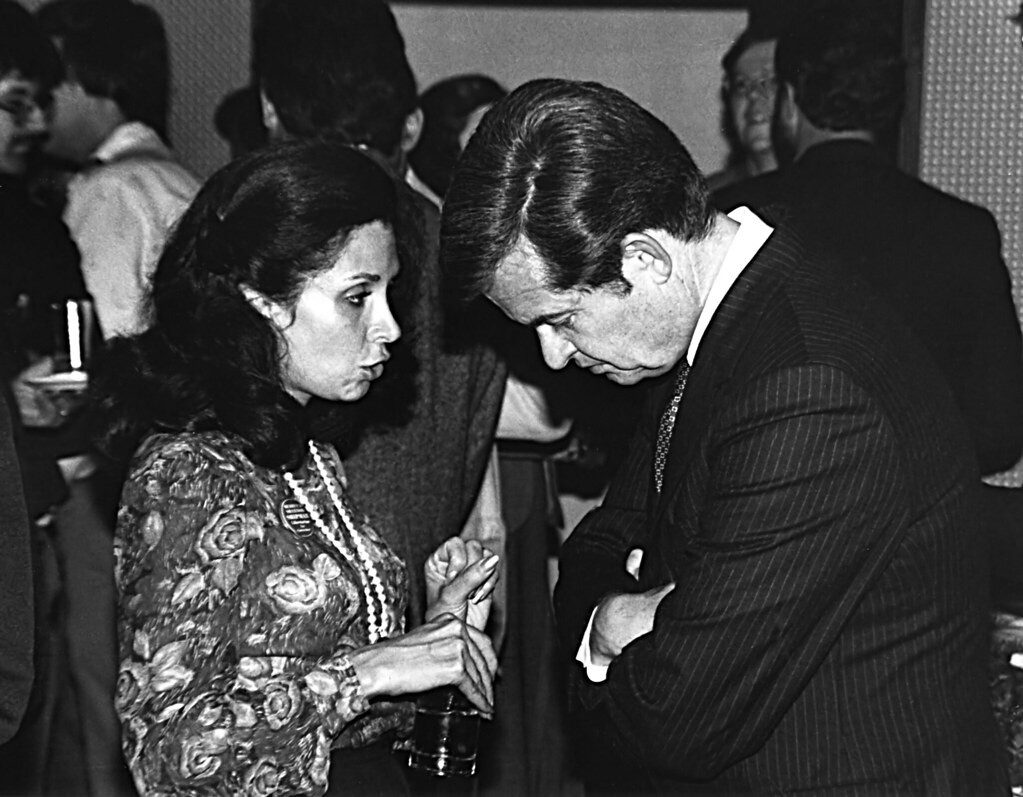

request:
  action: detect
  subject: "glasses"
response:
[723,73,777,98]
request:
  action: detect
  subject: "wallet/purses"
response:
[408,685,480,779]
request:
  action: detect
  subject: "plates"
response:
[26,375,89,392]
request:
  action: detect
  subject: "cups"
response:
[407,683,482,779]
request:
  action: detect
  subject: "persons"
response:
[701,1,1022,477]
[0,0,586,797]
[84,140,499,797]
[440,78,1013,797]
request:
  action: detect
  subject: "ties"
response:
[655,360,692,493]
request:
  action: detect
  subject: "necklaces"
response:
[281,440,390,644]
